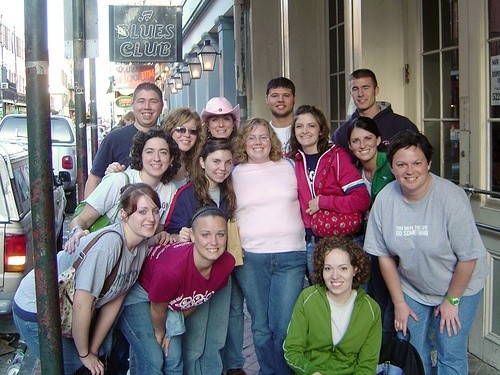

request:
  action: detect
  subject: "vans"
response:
[0,114,93,213]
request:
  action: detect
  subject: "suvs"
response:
[1,142,73,316]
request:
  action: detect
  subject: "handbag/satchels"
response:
[57,228,125,339]
[380,328,426,375]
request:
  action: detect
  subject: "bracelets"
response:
[79,351,89,358]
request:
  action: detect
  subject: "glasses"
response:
[246,135,272,144]
[170,127,200,135]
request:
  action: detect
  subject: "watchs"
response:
[446,295,461,305]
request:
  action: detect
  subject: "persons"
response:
[105,108,201,190]
[117,208,236,375]
[363,132,488,375]
[284,105,371,293]
[110,111,135,131]
[347,116,396,344]
[231,118,307,375]
[64,130,178,375]
[282,237,382,375]
[12,183,161,374]
[266,76,333,154]
[330,69,420,168]
[186,97,247,375]
[164,138,233,375]
[84,81,164,200]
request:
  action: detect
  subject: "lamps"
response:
[167,74,180,94]
[197,39,222,72]
[172,68,183,91]
[186,51,203,80]
[178,62,192,86]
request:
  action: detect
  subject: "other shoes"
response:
[226,368,249,375]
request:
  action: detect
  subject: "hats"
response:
[200,97,241,131]
